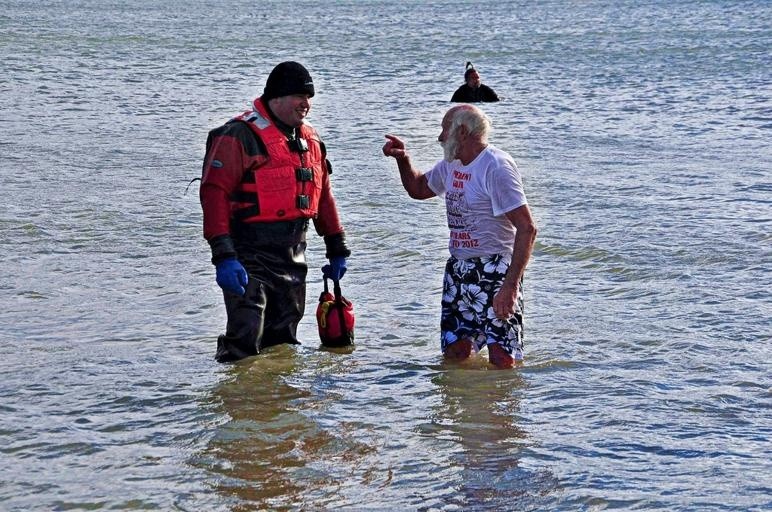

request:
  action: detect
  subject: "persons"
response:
[197,61,353,364]
[380,105,539,371]
[448,60,501,102]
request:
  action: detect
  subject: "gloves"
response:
[322,256,348,280]
[214,259,250,299]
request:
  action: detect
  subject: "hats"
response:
[262,60,315,101]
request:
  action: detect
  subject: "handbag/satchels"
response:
[315,274,355,348]
